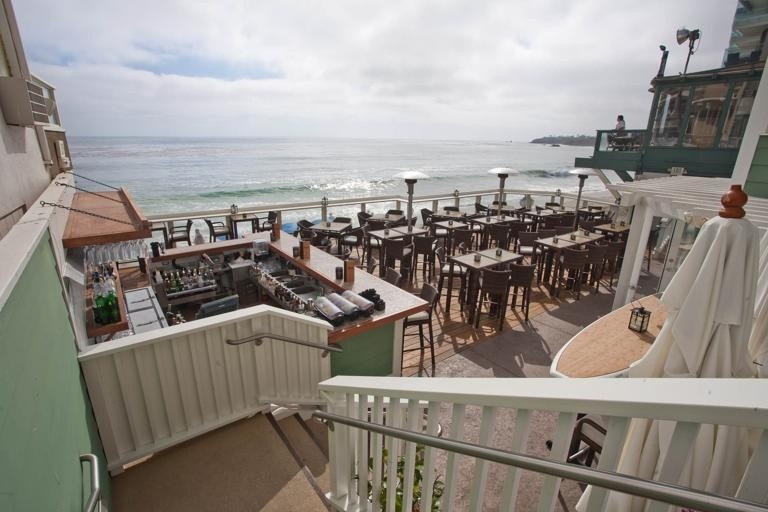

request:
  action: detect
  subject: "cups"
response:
[82,238,148,265]
[270,231,277,241]
[334,267,343,278]
[291,246,300,256]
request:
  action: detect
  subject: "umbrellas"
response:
[570,181,764,512]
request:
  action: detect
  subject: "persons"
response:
[328,213,334,220]
[612,113,626,150]
[192,228,206,245]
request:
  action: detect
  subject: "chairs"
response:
[544,413,608,468]
[606,128,647,151]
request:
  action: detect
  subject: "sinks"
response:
[212,263,231,274]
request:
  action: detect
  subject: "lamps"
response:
[677,28,699,75]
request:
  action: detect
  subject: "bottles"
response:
[92,270,120,320]
[250,265,318,318]
[163,269,217,292]
